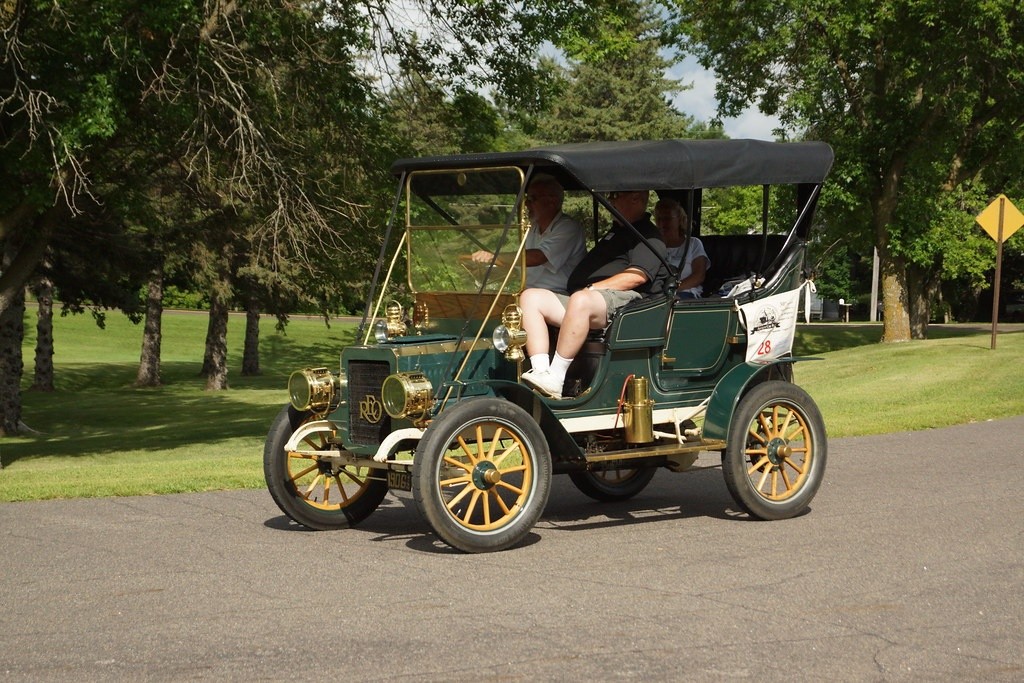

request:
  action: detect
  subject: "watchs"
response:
[586,284,594,290]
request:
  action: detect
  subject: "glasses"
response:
[653,215,677,223]
[523,193,546,204]
[607,192,630,200]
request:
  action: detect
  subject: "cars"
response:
[261,134,836,554]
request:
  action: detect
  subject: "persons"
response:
[654,201,710,301]
[519,189,667,400]
[471,174,585,298]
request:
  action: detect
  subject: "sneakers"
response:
[521,368,564,401]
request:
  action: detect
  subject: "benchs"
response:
[551,233,803,355]
